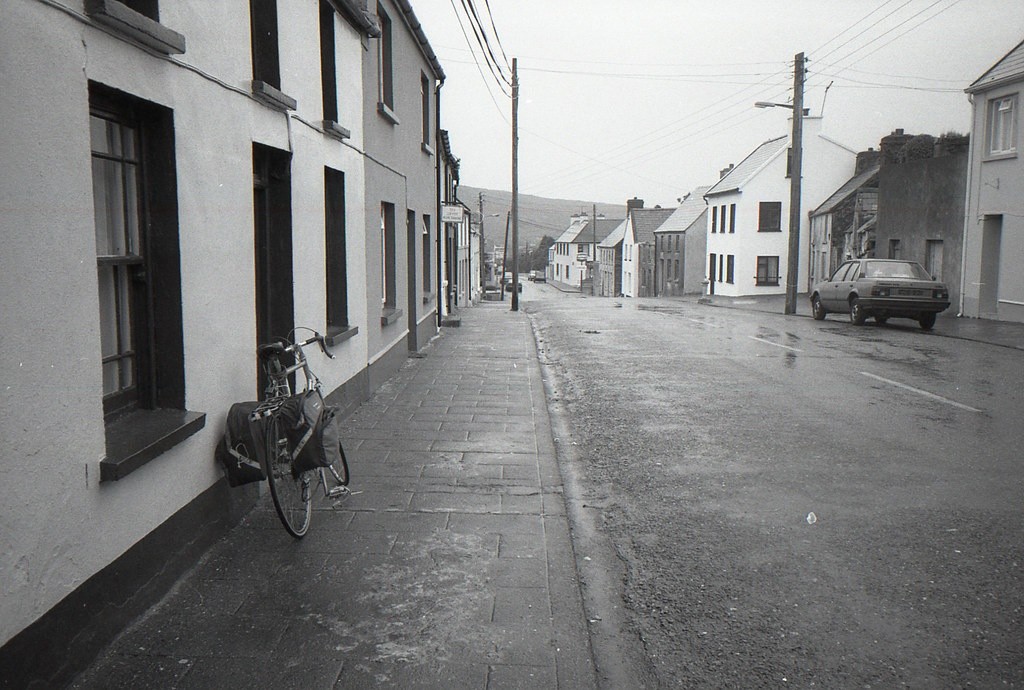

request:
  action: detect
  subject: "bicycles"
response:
[257,326,350,540]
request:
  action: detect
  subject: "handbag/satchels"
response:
[215,401,266,488]
[279,390,339,474]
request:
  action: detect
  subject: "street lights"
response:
[753,99,801,315]
[479,213,499,299]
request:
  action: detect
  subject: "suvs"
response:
[535,271,546,283]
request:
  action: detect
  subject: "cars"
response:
[528,269,536,281]
[506,279,522,292]
[810,257,952,329]
[500,272,513,284]
